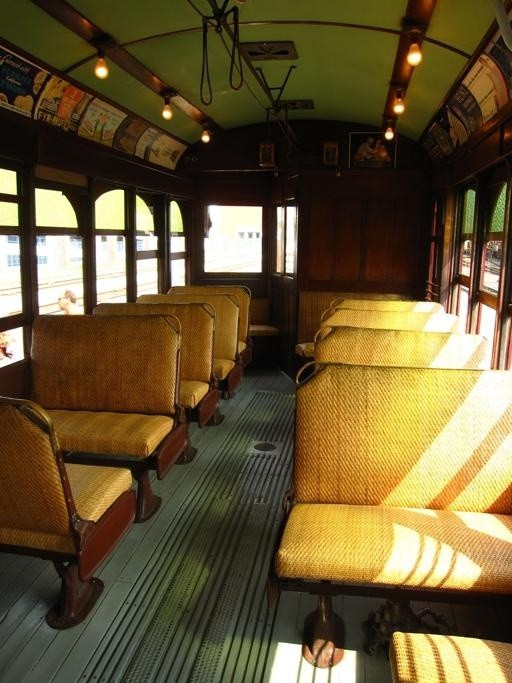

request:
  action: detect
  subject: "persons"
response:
[57,289,84,315]
[0,328,14,362]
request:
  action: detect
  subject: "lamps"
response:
[198,117,215,143]
[157,86,178,122]
[391,81,407,115]
[93,41,113,82]
[383,118,395,141]
[404,26,423,67]
